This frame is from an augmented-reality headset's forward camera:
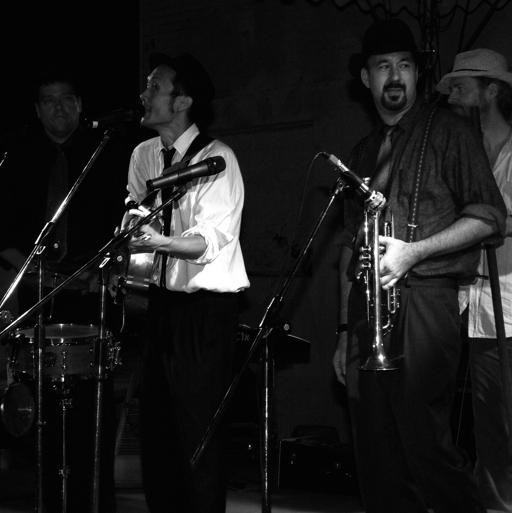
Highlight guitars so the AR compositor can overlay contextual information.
[114,161,186,315]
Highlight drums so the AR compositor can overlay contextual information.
[10,322,122,388]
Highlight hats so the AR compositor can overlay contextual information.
[349,19,438,71]
[435,48,512,95]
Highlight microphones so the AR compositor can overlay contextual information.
[146,156,226,192]
[90,104,145,129]
[325,153,388,211]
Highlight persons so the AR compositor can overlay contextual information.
[109,50,253,512]
[436,45,512,512]
[328,20,512,511]
[1,63,130,513]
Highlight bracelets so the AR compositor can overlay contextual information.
[334,322,349,335]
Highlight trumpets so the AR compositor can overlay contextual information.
[355,177,401,372]
